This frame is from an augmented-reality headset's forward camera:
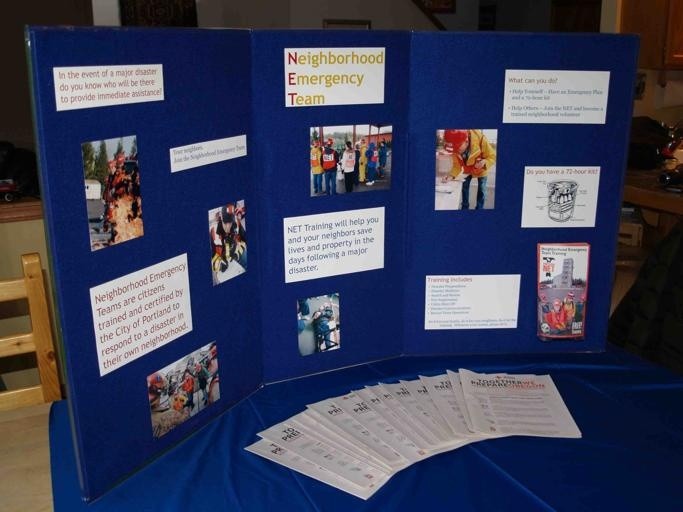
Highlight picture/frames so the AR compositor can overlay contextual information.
[119,0,198,26]
[422,0,456,14]
[322,19,371,28]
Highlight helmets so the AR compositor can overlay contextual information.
[147,369,169,406]
[359,139,366,144]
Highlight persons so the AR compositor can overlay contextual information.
[101,152,139,239]
[311,303,333,353]
[562,289,576,330]
[182,372,197,410]
[210,203,246,272]
[546,298,566,333]
[194,362,209,404]
[207,352,219,406]
[440,129,496,212]
[146,371,184,438]
[309,133,386,198]
[573,294,584,324]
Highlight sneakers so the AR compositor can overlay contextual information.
[365,180,375,186]
[313,190,324,195]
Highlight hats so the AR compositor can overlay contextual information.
[326,138,334,145]
[221,204,235,224]
[106,160,117,169]
[567,292,575,299]
[313,140,320,147]
[116,154,124,165]
[195,364,201,371]
[210,346,217,360]
[443,129,467,154]
[553,298,561,311]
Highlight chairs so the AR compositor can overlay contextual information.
[0,251,62,411]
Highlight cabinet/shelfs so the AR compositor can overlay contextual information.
[621,0,683,70]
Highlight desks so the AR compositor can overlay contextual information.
[0,197,64,392]
[622,169,683,246]
[1,343,682,511]
